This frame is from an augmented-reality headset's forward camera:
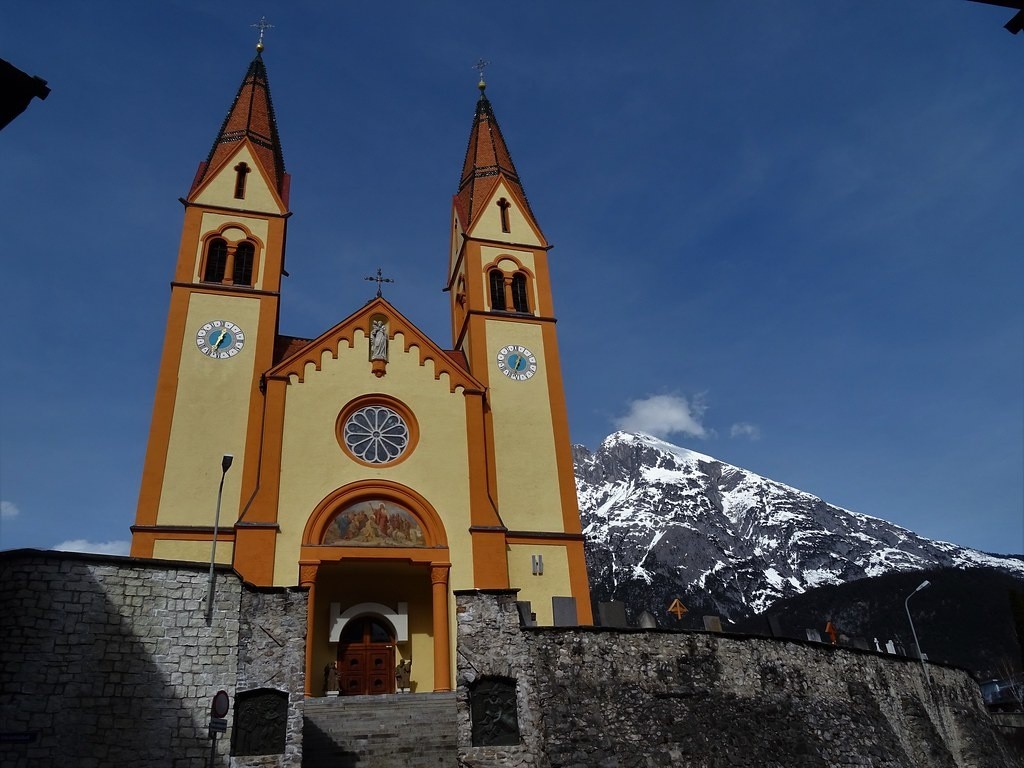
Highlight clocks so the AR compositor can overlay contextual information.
[497,345,537,380]
[196,320,245,358]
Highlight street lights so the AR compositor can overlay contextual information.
[905,579,938,700]
[203,455,233,618]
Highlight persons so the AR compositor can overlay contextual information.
[324,663,343,691]
[394,659,412,688]
[371,321,386,358]
